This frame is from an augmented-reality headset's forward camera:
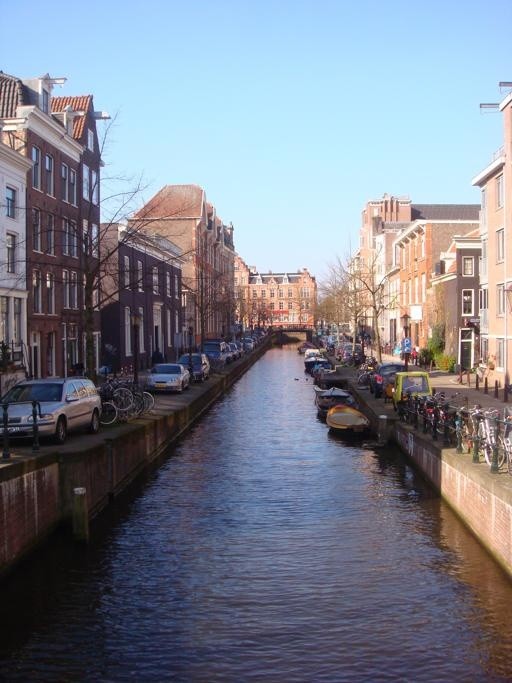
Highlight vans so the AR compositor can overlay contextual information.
[200,340,227,366]
[0,374,102,441]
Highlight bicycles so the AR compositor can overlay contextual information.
[399,390,512,475]
[357,356,379,383]
[91,373,155,425]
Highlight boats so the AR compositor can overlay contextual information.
[326,403,371,435]
[317,387,359,416]
[299,339,348,387]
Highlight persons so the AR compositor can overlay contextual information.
[154,346,164,363]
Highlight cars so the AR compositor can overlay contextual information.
[371,362,406,398]
[180,352,209,381]
[392,371,432,410]
[145,362,190,392]
[227,325,273,362]
[316,328,364,363]
